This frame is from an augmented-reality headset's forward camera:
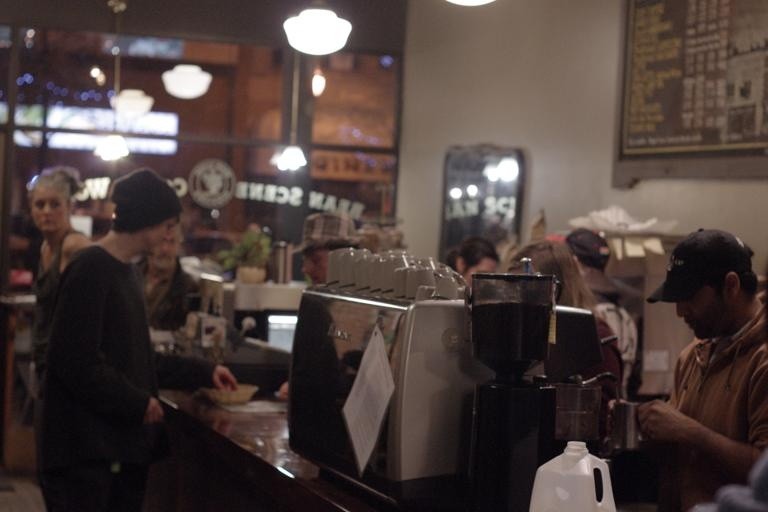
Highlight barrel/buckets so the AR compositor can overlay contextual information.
[526,439,618,512]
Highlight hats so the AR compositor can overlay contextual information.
[108,171,182,232]
[565,228,611,270]
[290,213,361,255]
[646,228,754,305]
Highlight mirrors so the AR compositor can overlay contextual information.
[438,144,523,268]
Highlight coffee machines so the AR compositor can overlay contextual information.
[458,270,557,512]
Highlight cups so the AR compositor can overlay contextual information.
[325,247,468,302]
[607,398,650,452]
